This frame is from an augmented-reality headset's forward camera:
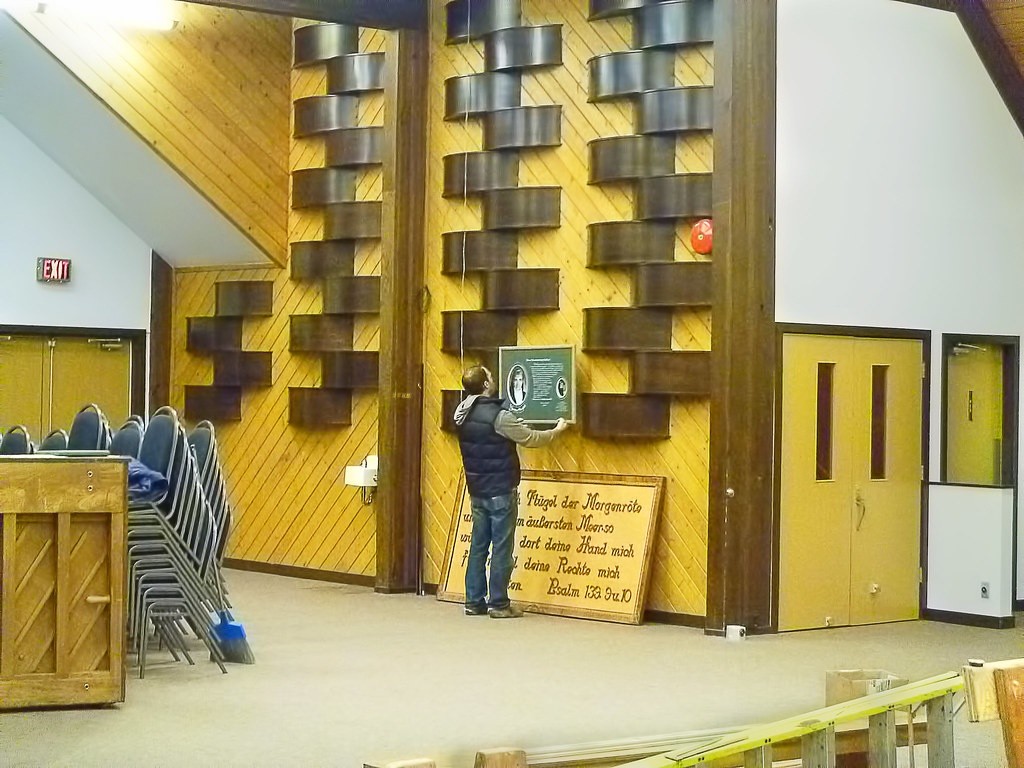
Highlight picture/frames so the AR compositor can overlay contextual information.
[435,462,668,625]
[500,344,578,428]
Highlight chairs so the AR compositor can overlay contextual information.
[0,404,236,679]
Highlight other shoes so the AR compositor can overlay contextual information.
[465,602,490,616]
[488,607,524,618]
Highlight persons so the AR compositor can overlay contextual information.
[509,369,526,403]
[453,365,570,619]
